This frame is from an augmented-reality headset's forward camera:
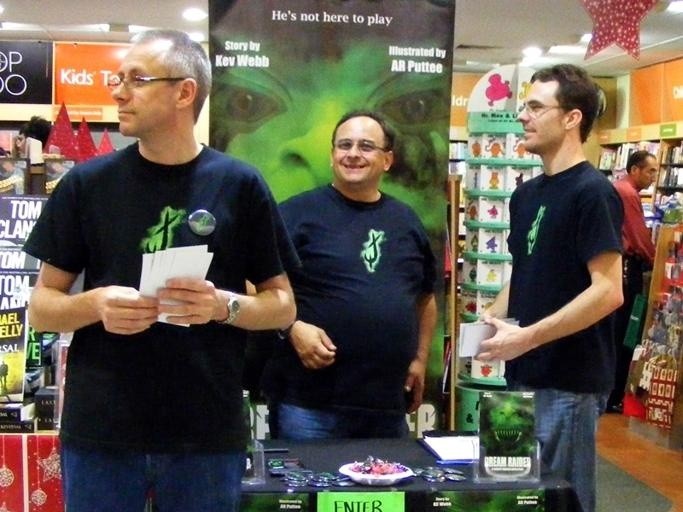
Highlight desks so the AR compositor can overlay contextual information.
[242,435,571,512]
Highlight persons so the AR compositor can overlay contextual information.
[471,62,626,512]
[21,29,297,510]
[611,149,656,348]
[268,107,440,441]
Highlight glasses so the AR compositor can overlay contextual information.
[518,101,571,115]
[333,137,386,152]
[107,69,185,91]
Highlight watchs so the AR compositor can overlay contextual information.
[215,287,241,325]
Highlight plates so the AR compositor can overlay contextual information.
[339,462,414,486]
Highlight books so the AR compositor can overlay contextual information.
[663,140,683,188]
